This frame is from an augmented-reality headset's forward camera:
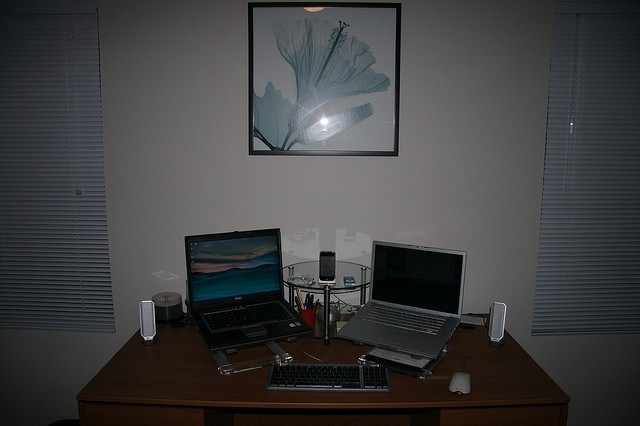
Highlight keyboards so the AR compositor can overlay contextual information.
[266,363,392,393]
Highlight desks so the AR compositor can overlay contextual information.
[283,259,372,347]
[75,312,571,425]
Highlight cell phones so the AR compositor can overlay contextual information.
[319,251,336,283]
[344,276,355,287]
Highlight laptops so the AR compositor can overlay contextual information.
[337,241,467,359]
[185,228,314,351]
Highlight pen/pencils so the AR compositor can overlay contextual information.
[295,287,319,309]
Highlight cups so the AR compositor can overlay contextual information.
[299,303,317,329]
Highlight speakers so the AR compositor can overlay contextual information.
[488,303,506,343]
[139,301,156,345]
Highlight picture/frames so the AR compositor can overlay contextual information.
[244,1,404,159]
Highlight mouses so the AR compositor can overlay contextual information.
[449,372,471,394]
